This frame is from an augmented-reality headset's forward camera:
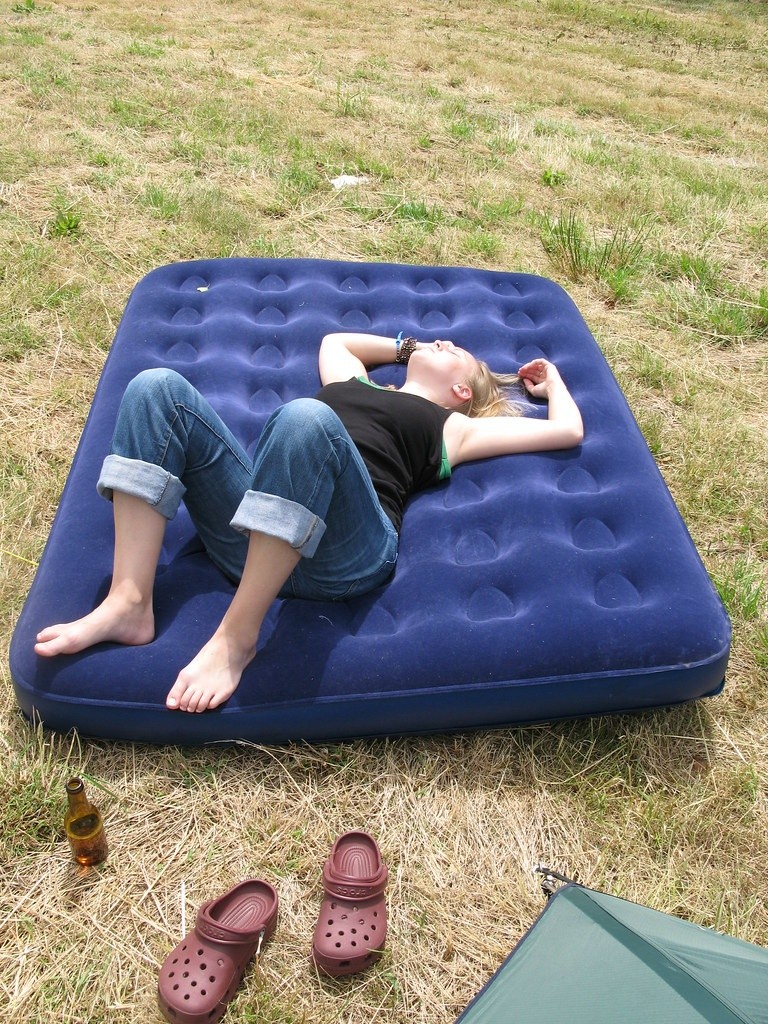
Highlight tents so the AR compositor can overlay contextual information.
[453,868,768,1024]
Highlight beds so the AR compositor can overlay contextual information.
[9,257,730,743]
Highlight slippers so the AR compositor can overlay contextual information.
[156,880,281,1022]
[311,829,390,976]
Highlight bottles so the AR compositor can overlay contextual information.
[64,777,108,866]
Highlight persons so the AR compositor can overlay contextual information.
[34,334,585,714]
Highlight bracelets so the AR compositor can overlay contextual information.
[397,331,418,364]
